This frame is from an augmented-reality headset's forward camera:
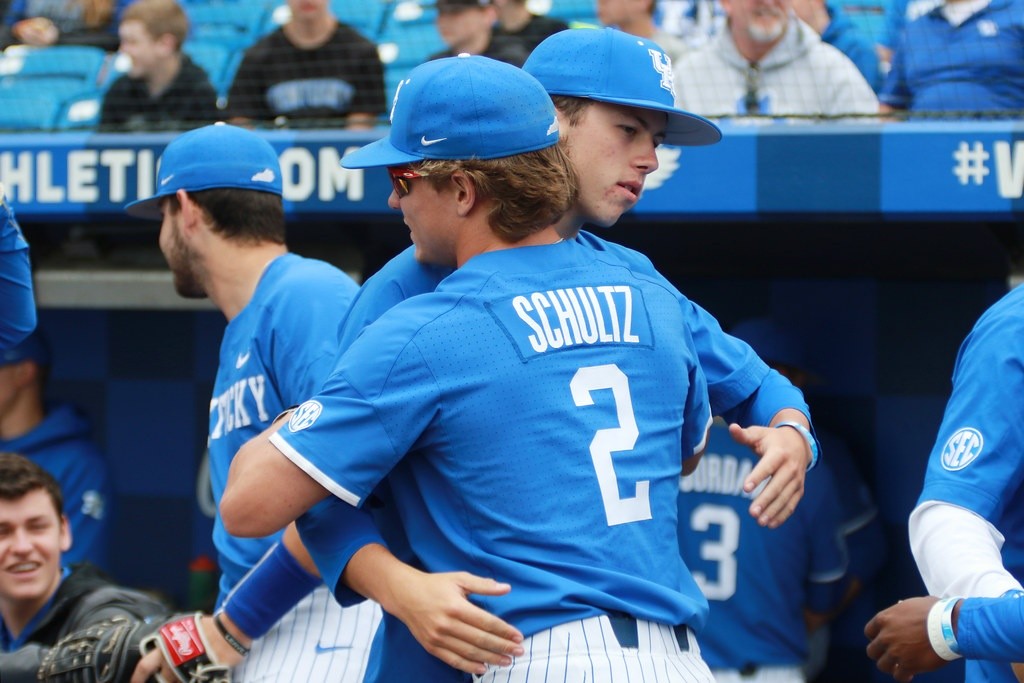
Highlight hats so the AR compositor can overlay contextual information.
[121,122,284,219]
[337,53,561,169]
[519,26,723,148]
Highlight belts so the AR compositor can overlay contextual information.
[605,613,690,653]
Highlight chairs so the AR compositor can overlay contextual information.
[0,0,893,112]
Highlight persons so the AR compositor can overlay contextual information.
[672,0,878,113]
[864,596,1024,683]
[124,123,383,683]
[0,0,119,52]
[0,451,167,683]
[790,0,1024,115]
[220,53,713,683]
[294,27,822,683]
[908,283,1024,683]
[0,180,37,345]
[0,332,112,580]
[597,0,690,64]
[100,0,220,134]
[677,319,886,682]
[222,0,387,132]
[425,0,570,68]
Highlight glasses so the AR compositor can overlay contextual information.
[384,168,455,196]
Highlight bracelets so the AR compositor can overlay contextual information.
[214,613,250,658]
[775,421,818,472]
[927,598,963,662]
